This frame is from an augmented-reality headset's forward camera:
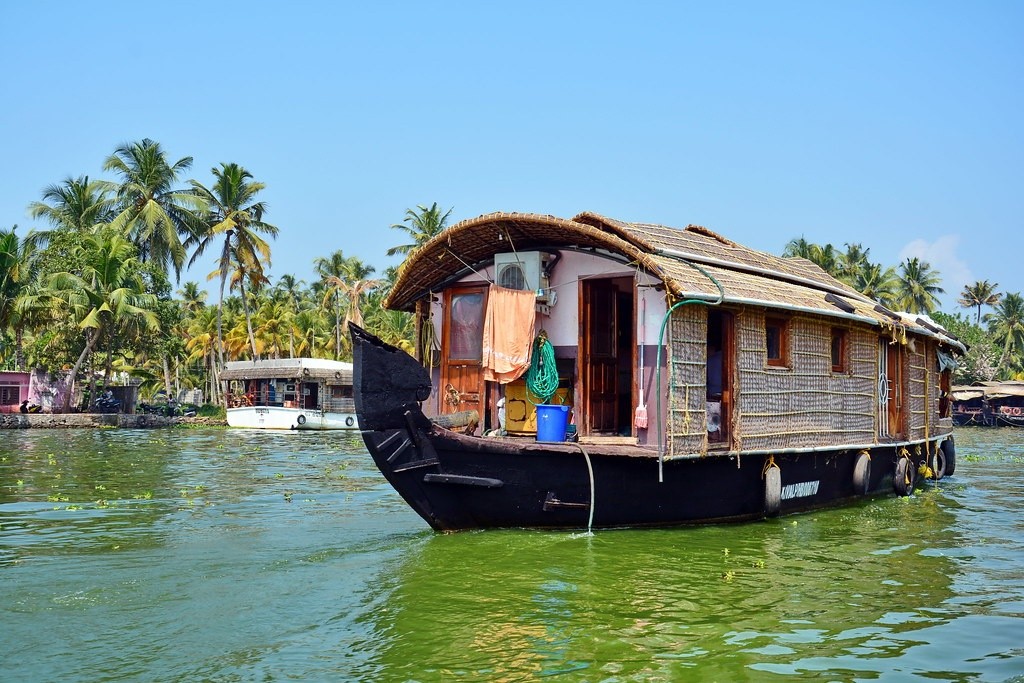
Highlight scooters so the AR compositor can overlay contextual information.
[89,397,122,413]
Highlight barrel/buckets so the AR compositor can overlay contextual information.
[535,404,571,442]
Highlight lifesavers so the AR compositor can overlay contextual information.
[764,466,782,518]
[345,417,354,426]
[239,395,248,407]
[230,397,241,408]
[1003,407,1012,415]
[939,439,956,477]
[297,415,306,425]
[852,453,871,500]
[248,394,256,406]
[893,457,916,498]
[1012,408,1021,416]
[928,447,946,480]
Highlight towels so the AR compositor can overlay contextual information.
[481,283,537,384]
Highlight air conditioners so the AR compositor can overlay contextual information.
[495,250,551,302]
[283,384,296,394]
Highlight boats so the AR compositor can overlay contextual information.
[220,357,359,430]
[347,211,971,533]
[950,380,1024,427]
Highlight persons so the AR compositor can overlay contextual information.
[940,390,956,402]
[165,393,176,418]
[100,386,116,408]
